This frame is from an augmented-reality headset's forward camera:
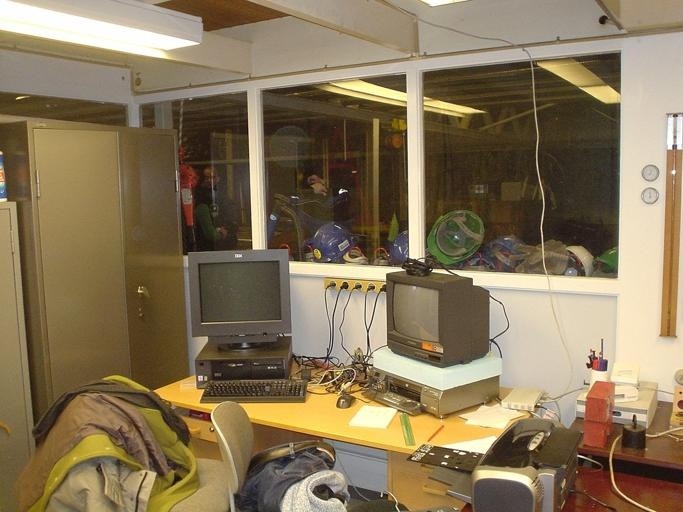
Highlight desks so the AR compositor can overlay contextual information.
[563,456,683,511]
[147,369,543,512]
[566,398,681,471]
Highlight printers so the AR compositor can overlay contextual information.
[406,418,585,512]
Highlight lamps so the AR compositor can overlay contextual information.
[315,81,488,119]
[1,0,204,59]
[538,59,620,105]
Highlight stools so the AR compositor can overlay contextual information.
[136,456,231,512]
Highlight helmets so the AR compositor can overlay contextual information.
[566,244,596,279]
[597,244,618,273]
[427,207,487,265]
[489,234,531,273]
[392,229,409,263]
[314,222,353,260]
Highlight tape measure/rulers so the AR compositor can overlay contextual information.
[400,413,416,445]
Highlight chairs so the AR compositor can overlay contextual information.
[210,400,255,512]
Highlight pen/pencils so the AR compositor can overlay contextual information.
[428,425,445,442]
[586,339,608,371]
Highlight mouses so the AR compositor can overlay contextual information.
[336,396,355,409]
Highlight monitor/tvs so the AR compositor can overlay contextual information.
[386,271,489,368]
[188,249,292,350]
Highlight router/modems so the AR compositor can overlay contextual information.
[501,388,544,411]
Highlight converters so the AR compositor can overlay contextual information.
[301,369,311,380]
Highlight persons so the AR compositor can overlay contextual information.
[190,164,239,251]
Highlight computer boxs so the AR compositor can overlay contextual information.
[361,349,503,419]
[194,336,292,389]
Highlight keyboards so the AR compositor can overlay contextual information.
[200,379,308,403]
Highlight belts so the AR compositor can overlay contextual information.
[249,436,340,470]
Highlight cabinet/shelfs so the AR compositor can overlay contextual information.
[0,200,38,512]
[0,119,185,419]
